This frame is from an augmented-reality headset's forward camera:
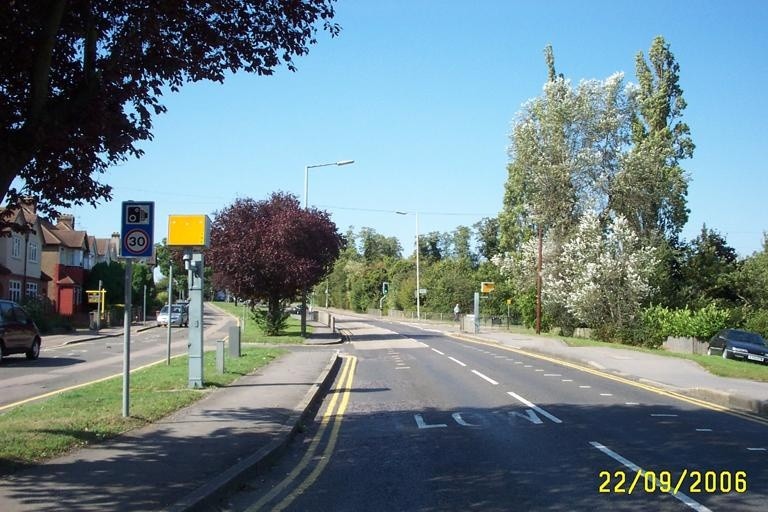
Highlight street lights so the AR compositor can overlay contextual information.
[297,159,355,338]
[394,210,421,320]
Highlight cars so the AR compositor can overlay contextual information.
[0,299,42,361]
[156,303,188,329]
[706,327,766,368]
[212,289,309,316]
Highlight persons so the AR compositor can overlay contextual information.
[452,304,461,322]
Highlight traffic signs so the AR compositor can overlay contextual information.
[123,228,151,255]
